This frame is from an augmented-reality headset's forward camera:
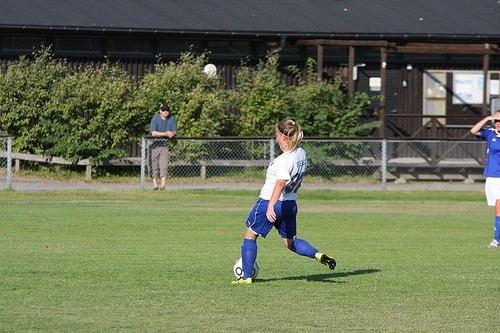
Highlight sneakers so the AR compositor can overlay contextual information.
[488,240,500,248]
[315,252,336,270]
[231,278,253,283]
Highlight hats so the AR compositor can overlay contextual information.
[159,103,169,109]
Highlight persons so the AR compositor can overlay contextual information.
[470,109,500,247]
[231,120,337,284]
[149,103,177,190]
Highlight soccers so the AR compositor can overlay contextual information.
[233,257,259,281]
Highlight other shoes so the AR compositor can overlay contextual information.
[154,186,158,190]
[160,186,164,190]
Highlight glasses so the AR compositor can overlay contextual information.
[161,109,169,111]
